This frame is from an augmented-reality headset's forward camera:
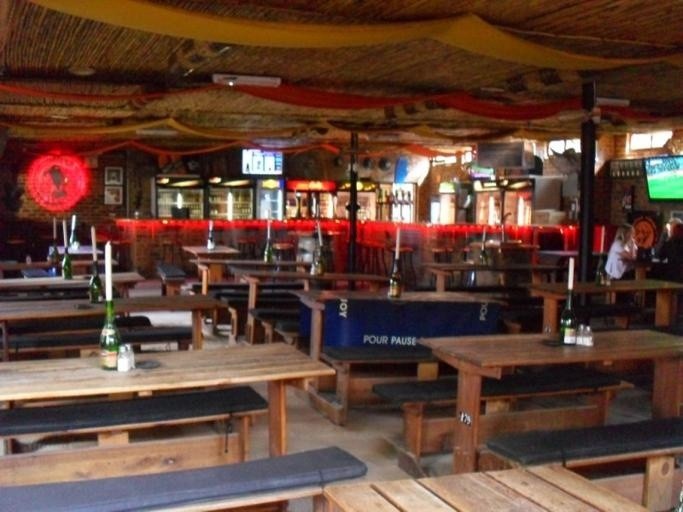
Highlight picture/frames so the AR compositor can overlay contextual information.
[103,165,123,186]
[102,185,124,206]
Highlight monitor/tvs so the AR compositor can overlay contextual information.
[241,148,285,177]
[642,155,683,203]
[476,142,524,169]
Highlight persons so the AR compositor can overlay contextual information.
[657,218,683,280]
[605,223,636,281]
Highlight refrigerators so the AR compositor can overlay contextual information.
[209,176,253,219]
[151,175,205,218]
[287,180,336,219]
[255,178,285,219]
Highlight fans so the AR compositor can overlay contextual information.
[544,147,582,195]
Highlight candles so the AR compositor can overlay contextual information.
[51,215,58,241]
[265,219,271,240]
[316,221,323,246]
[59,216,69,248]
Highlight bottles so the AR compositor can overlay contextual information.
[309,245,325,276]
[88,263,101,303]
[389,261,403,301]
[556,288,579,346]
[48,228,82,280]
[206,230,216,250]
[596,255,615,289]
[478,244,487,264]
[264,240,275,263]
[609,159,643,177]
[159,190,331,219]
[118,345,139,373]
[99,299,118,369]
[579,324,595,347]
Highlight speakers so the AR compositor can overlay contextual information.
[583,82,595,111]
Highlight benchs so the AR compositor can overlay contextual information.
[1,234,682,512]
